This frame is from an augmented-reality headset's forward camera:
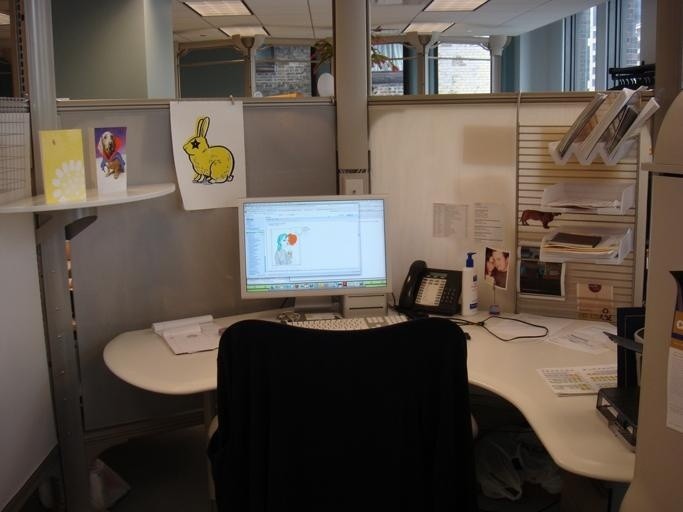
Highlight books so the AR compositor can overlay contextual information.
[556,88,659,162]
[541,226,627,261]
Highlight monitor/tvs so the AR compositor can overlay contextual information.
[239,195,388,319]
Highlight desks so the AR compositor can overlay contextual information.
[96,300,636,488]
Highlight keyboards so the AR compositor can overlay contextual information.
[286,315,406,331]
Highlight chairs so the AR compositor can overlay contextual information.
[208,315,485,508]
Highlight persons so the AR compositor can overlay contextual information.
[485,248,510,286]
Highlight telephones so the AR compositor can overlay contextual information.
[399,260,462,316]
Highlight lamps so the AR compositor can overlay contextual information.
[408,31,440,56]
[481,33,507,56]
[231,30,265,57]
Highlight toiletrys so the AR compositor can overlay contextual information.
[462,252,479,317]
